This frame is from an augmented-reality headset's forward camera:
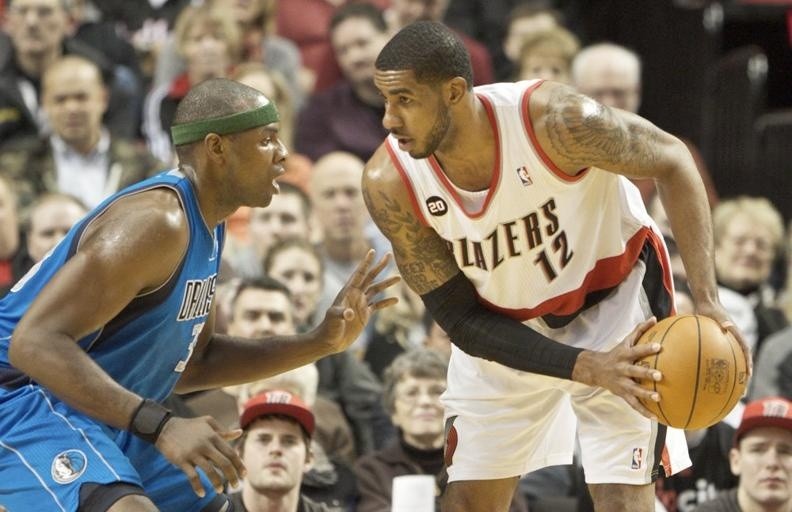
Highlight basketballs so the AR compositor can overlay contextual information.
[633,315,745,429]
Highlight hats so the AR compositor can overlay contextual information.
[239,389,315,436]
[734,396,792,450]
